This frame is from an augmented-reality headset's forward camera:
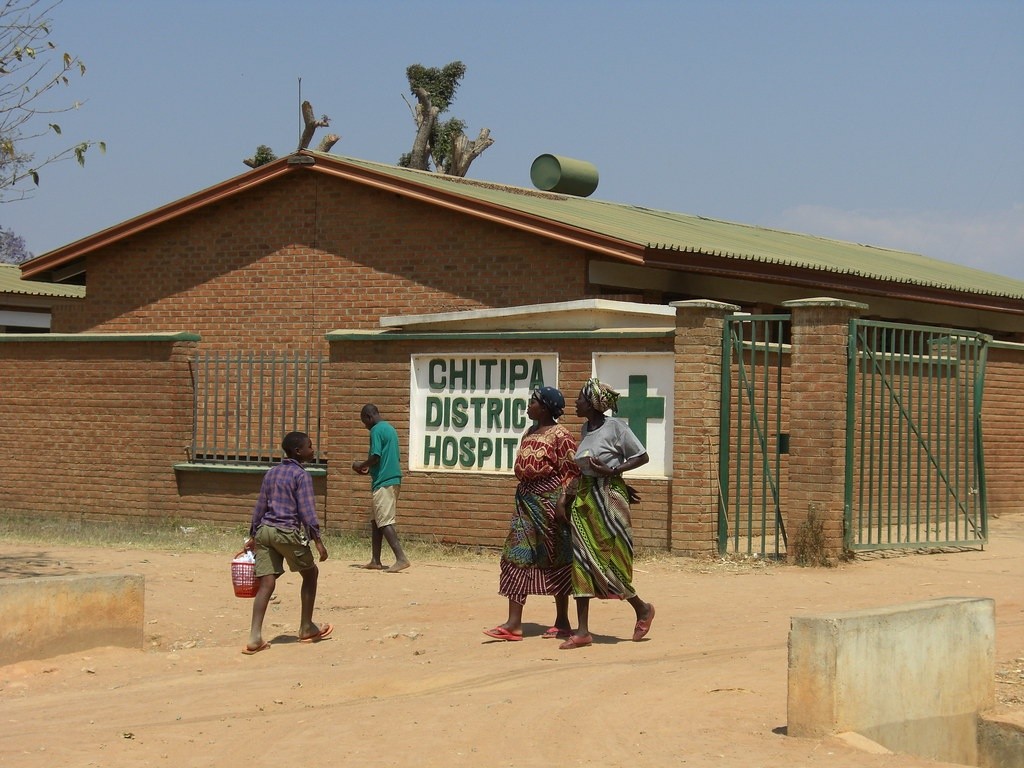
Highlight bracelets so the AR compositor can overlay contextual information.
[611,465,617,475]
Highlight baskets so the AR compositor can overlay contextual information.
[231,548,260,597]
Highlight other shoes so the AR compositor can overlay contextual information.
[560,631,593,649]
[633,603,655,641]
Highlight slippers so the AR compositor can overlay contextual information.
[483,625,523,641]
[298,621,334,642]
[242,639,271,655]
[543,625,573,638]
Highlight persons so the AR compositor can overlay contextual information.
[559,378,655,649]
[352,403,411,572]
[242,432,334,654]
[482,387,573,640]
